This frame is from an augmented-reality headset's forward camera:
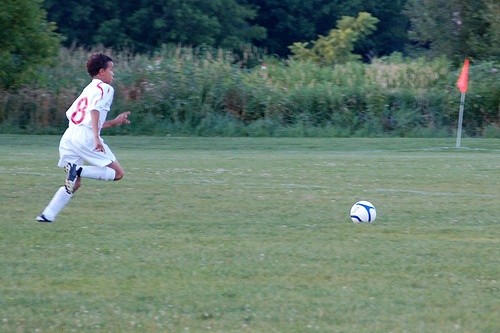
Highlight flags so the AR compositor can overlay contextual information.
[457,59,470,93]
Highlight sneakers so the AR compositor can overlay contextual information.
[64,162,78,194]
[36,214,52,223]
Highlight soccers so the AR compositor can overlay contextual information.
[349,200,377,225]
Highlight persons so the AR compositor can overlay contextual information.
[34,53,131,224]
